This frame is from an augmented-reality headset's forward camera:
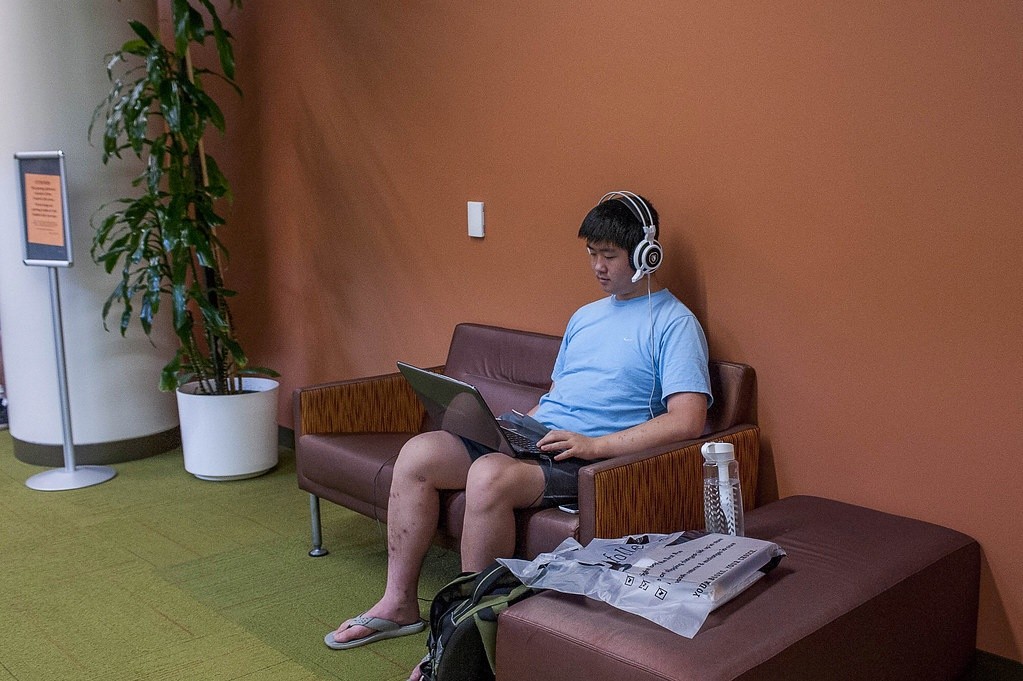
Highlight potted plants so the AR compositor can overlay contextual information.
[86,0,281,482]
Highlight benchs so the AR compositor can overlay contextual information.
[293,323,760,561]
[495,495,980,681]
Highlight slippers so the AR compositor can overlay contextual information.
[324,612,426,650]
[406,651,432,681]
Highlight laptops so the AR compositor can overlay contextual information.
[396,361,563,458]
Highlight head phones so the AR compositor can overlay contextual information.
[598,190,664,283]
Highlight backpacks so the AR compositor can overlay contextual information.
[427,562,559,681]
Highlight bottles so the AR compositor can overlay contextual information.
[700,440,744,537]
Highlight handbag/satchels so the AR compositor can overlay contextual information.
[494,531,787,638]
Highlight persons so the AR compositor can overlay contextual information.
[324,190,713,681]
